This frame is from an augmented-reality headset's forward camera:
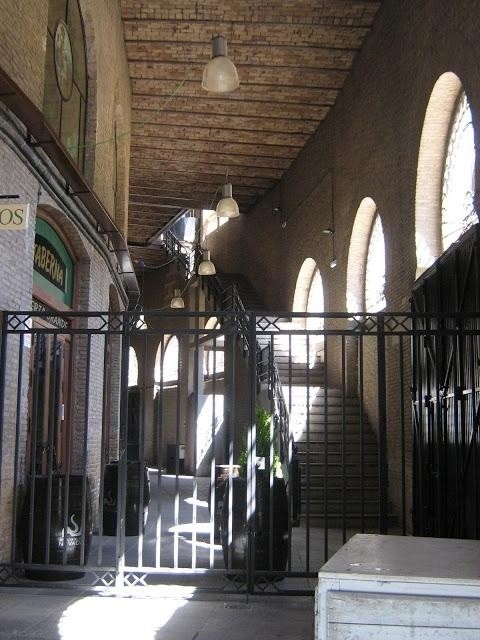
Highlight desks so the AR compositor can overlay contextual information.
[312,532,480,640]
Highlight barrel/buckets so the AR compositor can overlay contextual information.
[221,478,288,582]
[17,474,92,581]
[208,464,241,538]
[99,462,150,536]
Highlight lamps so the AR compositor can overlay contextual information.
[214,171,240,219]
[201,23,240,93]
[132,262,149,330]
[170,257,186,310]
[196,239,217,276]
